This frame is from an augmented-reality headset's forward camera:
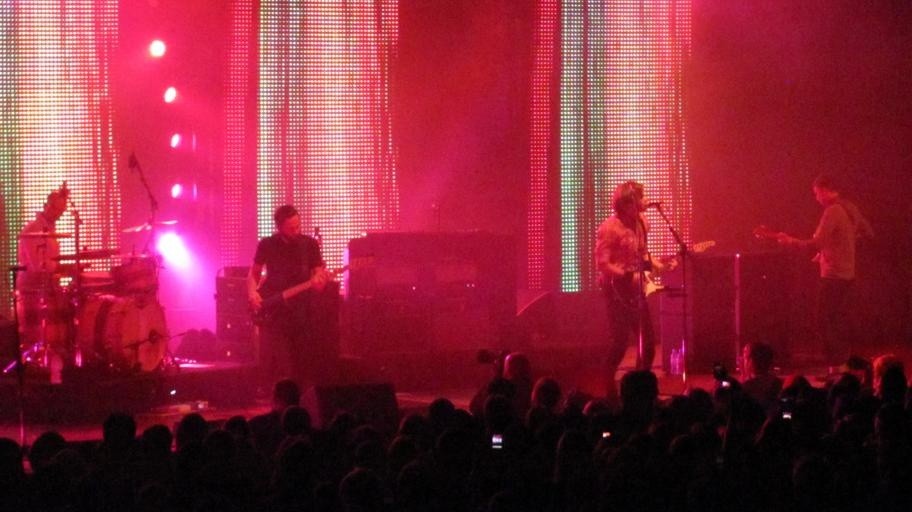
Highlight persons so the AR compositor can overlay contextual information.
[773,175,879,363]
[246,204,338,379]
[17,192,69,334]
[1,340,912,512]
[595,180,676,391]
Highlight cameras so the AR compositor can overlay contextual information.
[475,348,510,368]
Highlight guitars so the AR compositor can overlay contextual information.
[250,259,353,327]
[753,225,881,281]
[603,241,715,311]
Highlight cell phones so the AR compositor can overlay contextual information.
[603,431,611,438]
[781,410,791,422]
[491,430,502,449]
[713,362,727,378]
[721,379,730,388]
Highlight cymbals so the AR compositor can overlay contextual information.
[111,251,153,258]
[92,248,120,255]
[122,219,177,233]
[17,231,70,238]
[51,248,111,260]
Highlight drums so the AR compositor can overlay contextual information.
[80,292,168,375]
[70,271,115,299]
[110,263,159,293]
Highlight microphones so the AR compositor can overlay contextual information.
[313,224,322,236]
[645,201,663,210]
